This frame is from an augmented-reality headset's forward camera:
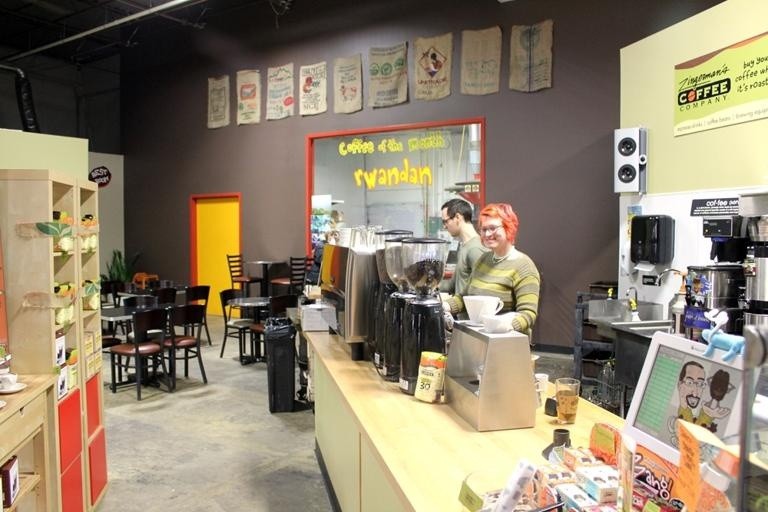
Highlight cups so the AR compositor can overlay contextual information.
[350,224,386,251]
[554,429,571,448]
[556,377,581,423]
[0,371,19,390]
[463,295,504,323]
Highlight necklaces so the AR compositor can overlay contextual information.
[492,245,514,264]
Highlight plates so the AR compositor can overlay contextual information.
[0,400,7,408]
[0,382,29,394]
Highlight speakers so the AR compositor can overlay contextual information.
[611,127,647,196]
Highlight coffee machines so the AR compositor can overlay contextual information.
[370,228,451,395]
[739,192,768,314]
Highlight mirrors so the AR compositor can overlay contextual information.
[305,117,485,269]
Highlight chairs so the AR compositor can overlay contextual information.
[101,252,305,400]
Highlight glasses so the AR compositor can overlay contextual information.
[479,224,503,236]
[442,217,451,226]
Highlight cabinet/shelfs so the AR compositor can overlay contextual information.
[0,168,109,512]
[0,373,54,512]
[574,281,617,397]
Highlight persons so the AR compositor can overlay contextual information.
[444,202,541,347]
[439,200,489,295]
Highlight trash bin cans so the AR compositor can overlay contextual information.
[263,317,297,413]
